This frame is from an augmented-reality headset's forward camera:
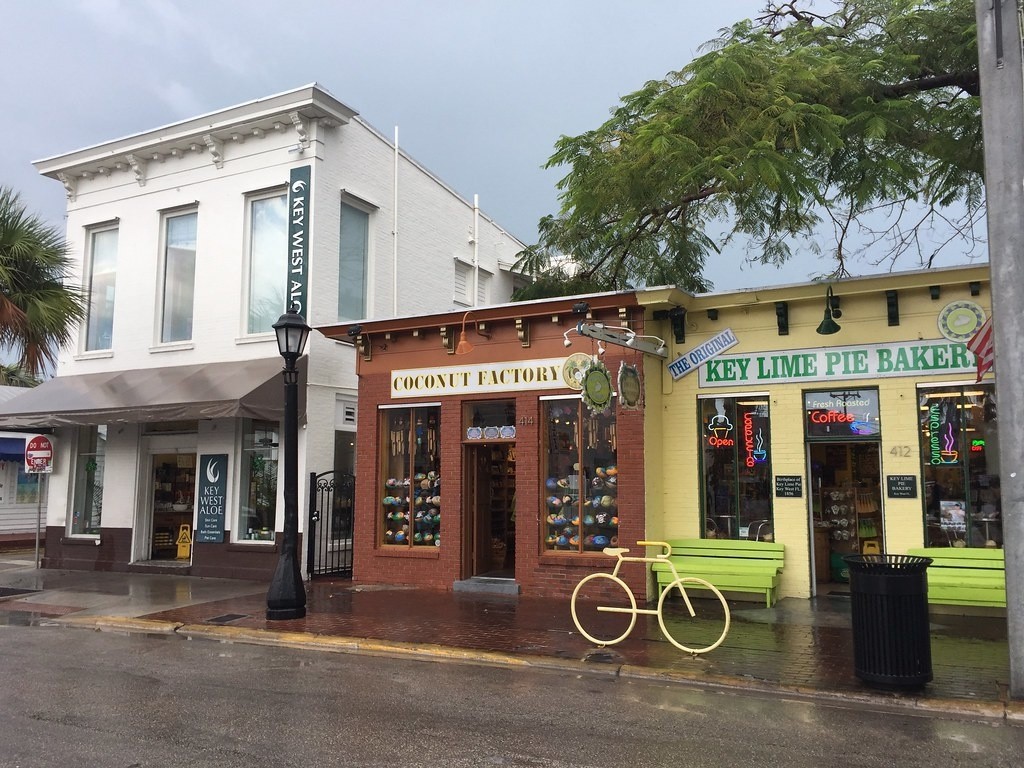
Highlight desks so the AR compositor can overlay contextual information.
[971,517,999,541]
[709,514,744,539]
[927,524,958,547]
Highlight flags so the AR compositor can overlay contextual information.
[966,315,993,384]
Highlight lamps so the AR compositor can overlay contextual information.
[347,325,365,336]
[563,323,666,356]
[817,285,841,336]
[572,302,592,314]
[455,312,492,355]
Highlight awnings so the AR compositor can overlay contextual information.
[0,354,307,430]
[0,437,26,463]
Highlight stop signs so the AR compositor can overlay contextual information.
[25,435,54,474]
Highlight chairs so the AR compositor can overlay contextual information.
[746,519,772,541]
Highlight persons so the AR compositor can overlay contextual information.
[975,478,1004,549]
[948,502,965,522]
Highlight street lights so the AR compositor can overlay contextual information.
[266,302,312,621]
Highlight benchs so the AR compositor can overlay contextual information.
[907,547,1006,609]
[651,539,785,608]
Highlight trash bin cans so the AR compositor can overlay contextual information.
[841,554,935,692]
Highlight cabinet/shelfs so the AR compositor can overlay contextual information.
[548,491,578,546]
[385,486,410,540]
[584,487,618,548]
[855,488,884,556]
[413,487,441,542]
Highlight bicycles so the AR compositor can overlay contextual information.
[571,541,730,653]
